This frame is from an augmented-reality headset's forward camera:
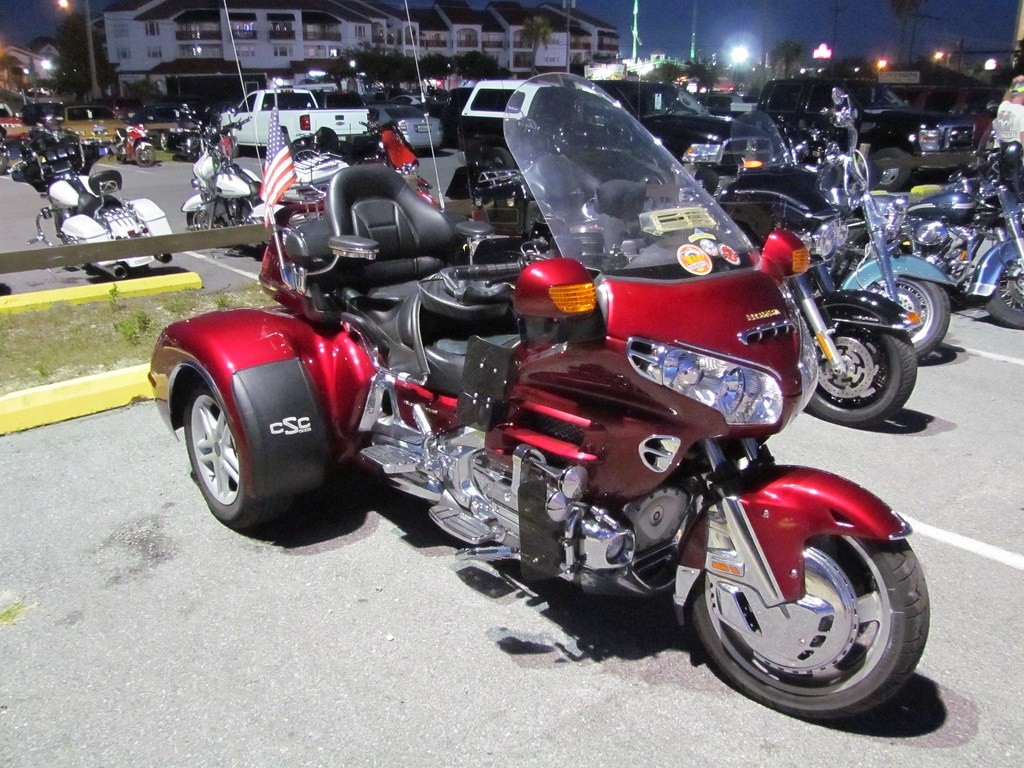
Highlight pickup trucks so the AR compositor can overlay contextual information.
[220,88,370,157]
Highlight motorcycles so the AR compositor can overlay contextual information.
[148,72,930,721]
[0,103,239,176]
[180,105,436,259]
[8,138,174,278]
[472,87,1024,430]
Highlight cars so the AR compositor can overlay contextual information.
[0,103,131,141]
[356,105,443,149]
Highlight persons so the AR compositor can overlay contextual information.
[975,33,1024,174]
[684,60,714,98]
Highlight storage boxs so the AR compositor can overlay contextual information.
[59,213,113,269]
[127,195,174,236]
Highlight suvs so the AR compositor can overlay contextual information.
[757,78,976,192]
[457,79,773,172]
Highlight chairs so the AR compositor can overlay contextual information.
[326,165,456,302]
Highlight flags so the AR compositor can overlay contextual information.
[258,106,297,230]
